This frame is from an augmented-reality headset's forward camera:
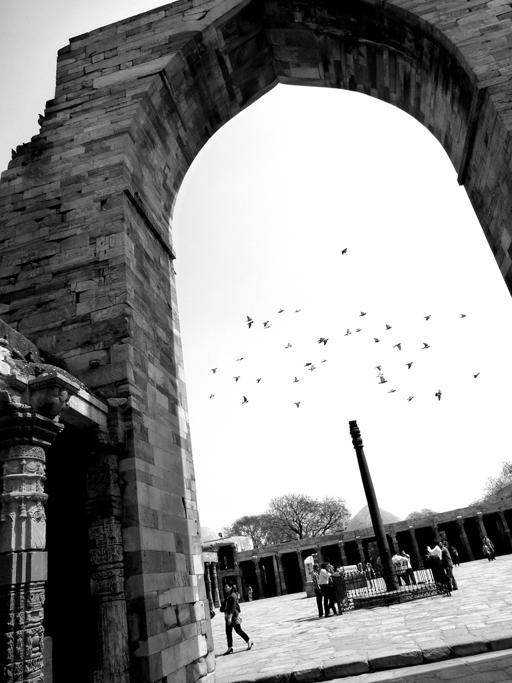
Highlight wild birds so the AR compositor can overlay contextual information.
[459,314,466,318]
[246,316,270,329]
[278,308,301,313]
[210,357,262,404]
[285,338,331,408]
[473,372,480,379]
[360,312,367,316]
[342,248,347,255]
[374,315,442,403]
[344,328,362,336]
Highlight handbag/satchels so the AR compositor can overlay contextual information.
[219,601,226,612]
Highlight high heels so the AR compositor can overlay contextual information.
[247,642,254,650]
[223,648,233,655]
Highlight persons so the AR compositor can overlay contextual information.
[230,583,241,604]
[480,535,496,560]
[481,543,494,562]
[220,582,255,655]
[311,538,461,616]
